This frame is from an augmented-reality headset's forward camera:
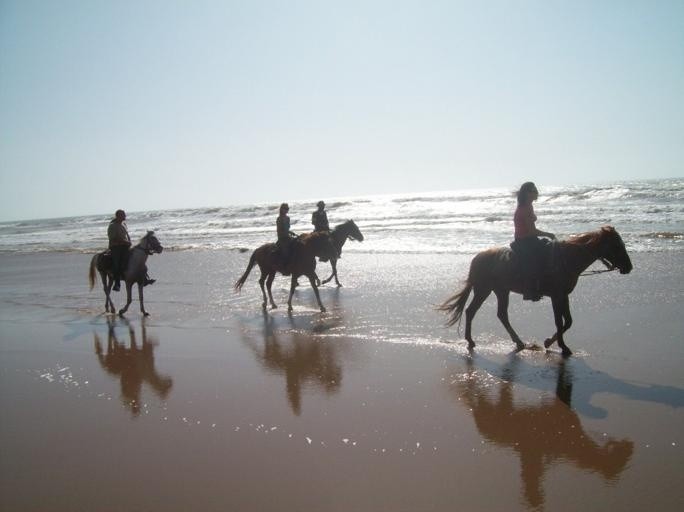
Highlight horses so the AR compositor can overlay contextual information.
[297,218,364,285]
[88,231,163,317]
[437,225,634,355]
[233,229,339,312]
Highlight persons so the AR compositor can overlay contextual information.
[312,201,329,231]
[276,203,295,243]
[107,210,155,291]
[515,183,555,300]
